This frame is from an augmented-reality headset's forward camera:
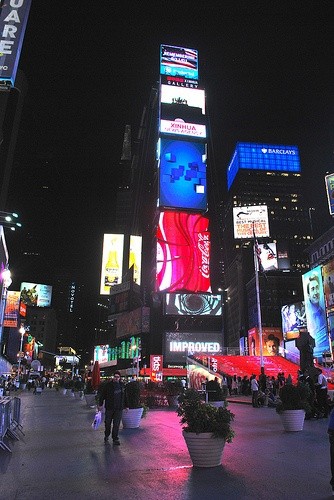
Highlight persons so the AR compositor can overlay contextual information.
[98,371,127,444]
[282,275,330,353]
[32,375,46,395]
[264,334,282,356]
[251,339,256,356]
[221,368,329,419]
[258,244,278,269]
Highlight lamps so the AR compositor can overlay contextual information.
[0,210,21,231]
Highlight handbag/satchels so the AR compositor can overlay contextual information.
[91,411,101,430]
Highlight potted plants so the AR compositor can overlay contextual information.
[276,381,313,431]
[176,396,237,468]
[167,381,180,407]
[121,382,148,429]
[58,379,97,406]
[207,380,225,408]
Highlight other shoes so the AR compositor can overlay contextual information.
[113,440,121,445]
[104,437,108,441]
